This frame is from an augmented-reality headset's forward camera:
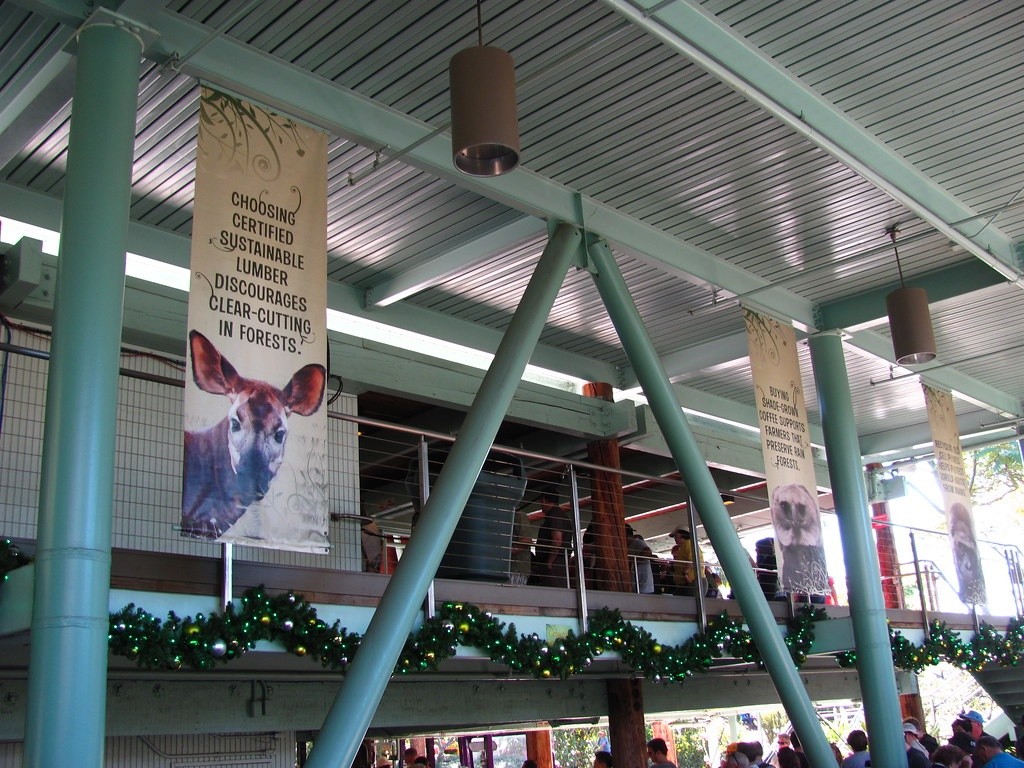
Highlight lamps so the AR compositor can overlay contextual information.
[449,0,520,178]
[885,229,937,367]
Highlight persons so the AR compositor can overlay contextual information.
[595,731,610,752]
[930,711,1024,768]
[728,546,760,600]
[522,759,537,768]
[404,747,432,768]
[375,755,391,768]
[718,741,776,768]
[778,730,810,768]
[704,560,723,599]
[823,574,839,606]
[668,524,694,584]
[625,523,656,594]
[901,716,939,768]
[842,730,870,768]
[593,751,614,768]
[535,486,572,588]
[506,509,533,585]
[646,737,677,768]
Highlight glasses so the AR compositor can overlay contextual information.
[777,742,790,746]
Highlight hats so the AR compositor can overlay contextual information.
[598,731,604,734]
[669,525,689,537]
[959,710,984,723]
[904,723,917,735]
